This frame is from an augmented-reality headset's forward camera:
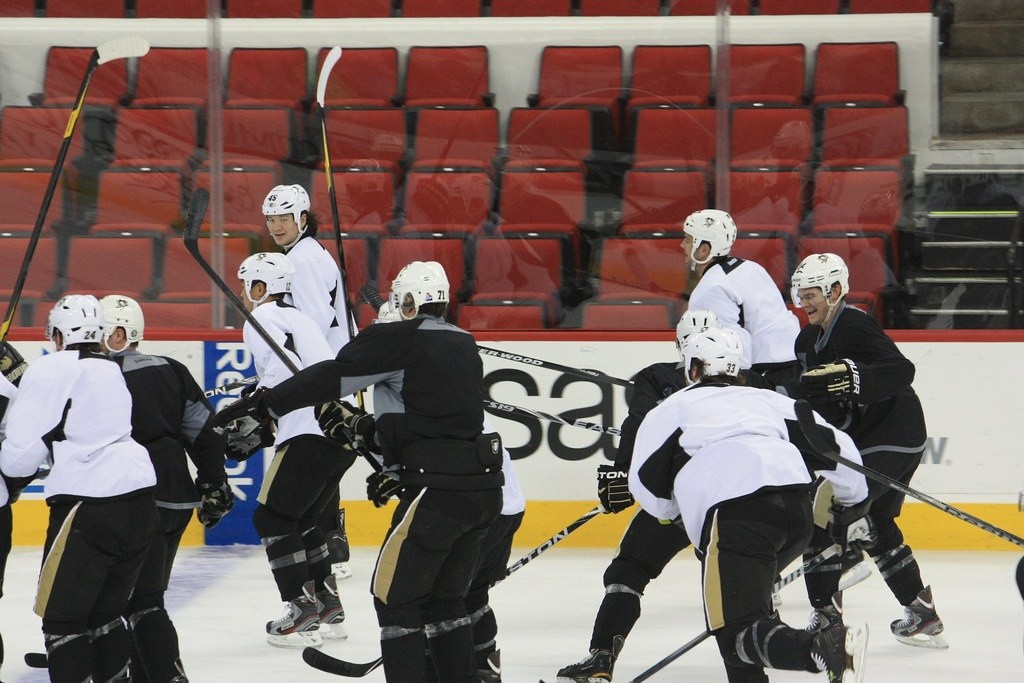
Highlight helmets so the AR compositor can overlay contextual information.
[676,308,721,350]
[683,209,737,265]
[790,252,849,308]
[682,328,743,384]
[100,295,144,352]
[262,184,311,233]
[48,294,105,350]
[391,260,450,319]
[237,253,293,304]
[378,301,402,323]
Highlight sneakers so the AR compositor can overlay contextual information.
[839,542,873,592]
[804,590,844,634]
[891,585,949,649]
[556,634,625,683]
[812,621,869,683]
[477,649,501,683]
[265,573,348,650]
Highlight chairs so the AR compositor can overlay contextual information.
[0,0,933,340]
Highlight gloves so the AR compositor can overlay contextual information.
[366,464,407,508]
[314,400,382,456]
[825,495,878,559]
[209,386,275,461]
[195,473,234,530]
[801,359,861,406]
[598,464,636,513]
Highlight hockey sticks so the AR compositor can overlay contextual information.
[1,36,152,346]
[24,652,48,669]
[314,43,367,415]
[302,503,607,681]
[180,187,404,499]
[791,396,1024,550]
[480,398,622,439]
[359,281,635,390]
[629,525,867,683]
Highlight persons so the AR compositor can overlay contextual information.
[225,183,526,636]
[0,294,234,683]
[210,260,506,683]
[680,209,802,400]
[559,252,945,683]
